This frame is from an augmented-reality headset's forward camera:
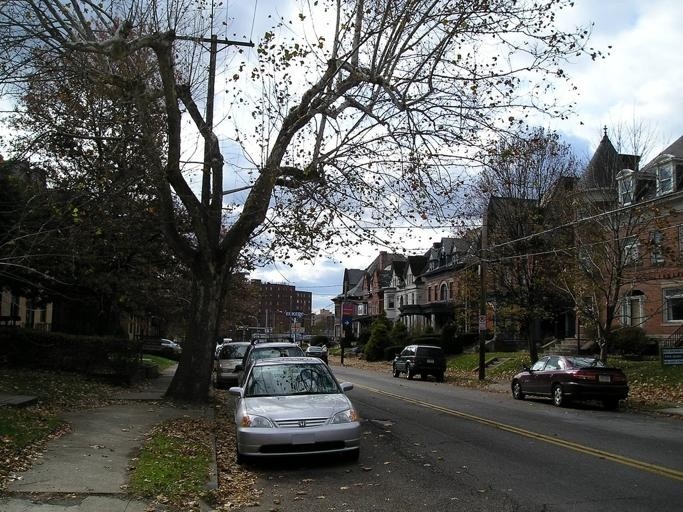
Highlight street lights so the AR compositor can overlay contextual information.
[249,315,260,331]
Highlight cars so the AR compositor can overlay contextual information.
[214,341,360,464]
[161,339,181,353]
[511,355,629,410]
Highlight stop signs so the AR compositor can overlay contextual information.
[344,303,354,316]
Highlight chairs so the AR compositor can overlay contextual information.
[249,371,279,395]
[293,370,320,392]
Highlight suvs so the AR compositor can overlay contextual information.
[392,345,446,382]
